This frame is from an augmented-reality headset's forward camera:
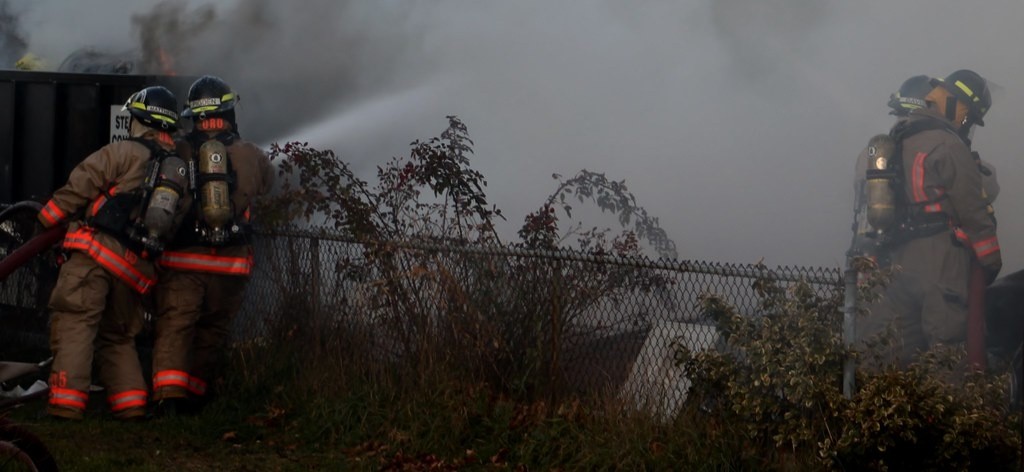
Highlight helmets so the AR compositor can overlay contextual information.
[930,67,994,128]
[181,75,241,116]
[128,86,181,132]
[889,75,944,114]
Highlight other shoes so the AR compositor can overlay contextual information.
[157,384,202,413]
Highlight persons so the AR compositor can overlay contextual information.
[29,85,185,420]
[845,67,1001,390]
[151,76,274,423]
